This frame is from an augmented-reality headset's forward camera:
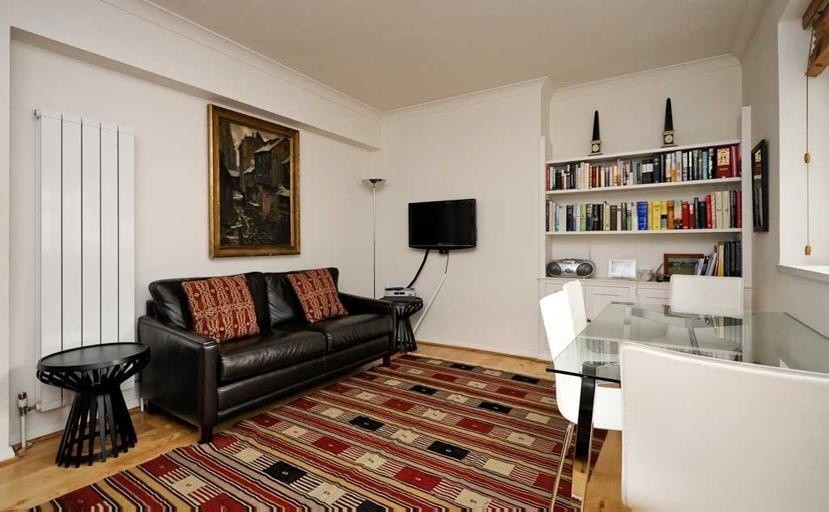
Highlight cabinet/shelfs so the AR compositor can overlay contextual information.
[539,284,635,358]
[635,284,670,319]
[543,139,742,283]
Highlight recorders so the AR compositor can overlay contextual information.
[547,259,596,278]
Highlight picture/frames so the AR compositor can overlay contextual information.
[663,253,705,276]
[750,138,771,233]
[608,258,637,280]
[205,100,302,260]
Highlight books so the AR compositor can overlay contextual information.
[544,145,741,192]
[545,187,743,232]
[696,239,742,276]
[700,313,743,345]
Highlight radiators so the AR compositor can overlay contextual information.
[30,107,136,411]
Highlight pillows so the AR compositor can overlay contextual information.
[181,275,262,341]
[284,266,349,325]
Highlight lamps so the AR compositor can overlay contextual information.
[362,178,386,299]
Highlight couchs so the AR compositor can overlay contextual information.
[137,264,397,444]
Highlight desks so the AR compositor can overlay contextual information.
[546,300,827,503]
[35,340,152,468]
[377,295,423,358]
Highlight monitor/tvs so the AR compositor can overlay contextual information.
[408,199,477,248]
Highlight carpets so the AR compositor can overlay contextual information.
[0,351,609,512]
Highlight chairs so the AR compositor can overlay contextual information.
[563,277,619,363]
[669,272,744,322]
[539,279,621,512]
[617,340,829,512]
